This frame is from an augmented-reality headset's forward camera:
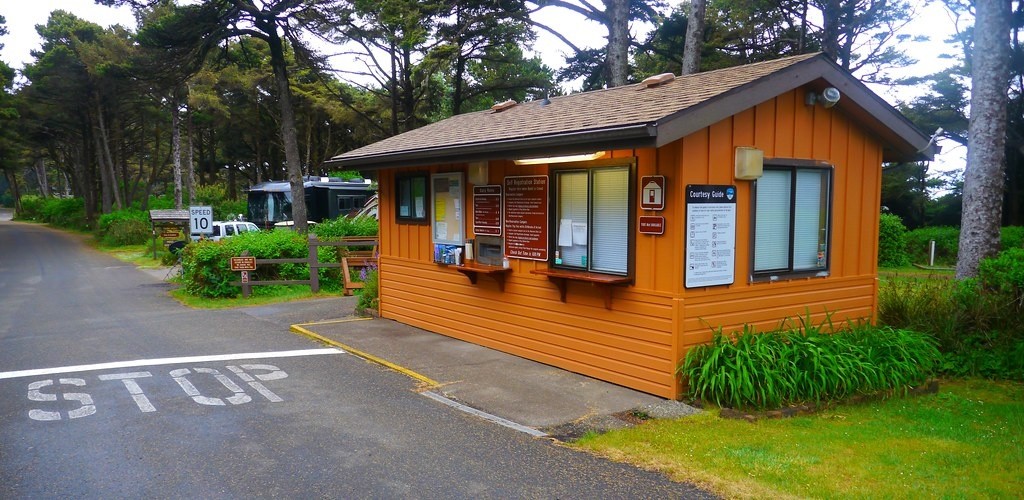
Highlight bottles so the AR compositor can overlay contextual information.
[502,255,509,268]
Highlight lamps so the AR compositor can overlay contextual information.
[513,151,605,165]
[805,87,840,108]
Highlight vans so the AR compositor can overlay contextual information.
[190,221,261,241]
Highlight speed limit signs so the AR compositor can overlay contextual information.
[189,206,213,234]
